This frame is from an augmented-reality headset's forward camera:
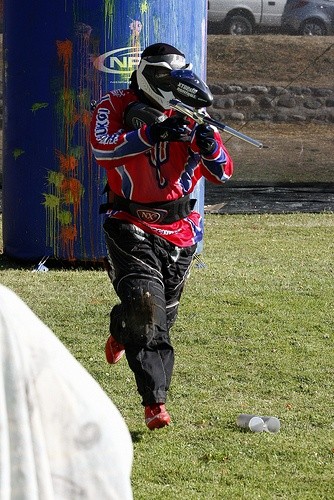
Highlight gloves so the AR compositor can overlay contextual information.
[145,116,192,143]
[195,123,216,156]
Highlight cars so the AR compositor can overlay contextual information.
[209,0,334,38]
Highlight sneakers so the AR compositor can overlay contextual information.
[105,334,125,364]
[144,404,170,430]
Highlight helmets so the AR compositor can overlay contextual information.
[137,43,185,109]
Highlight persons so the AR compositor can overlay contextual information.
[89,43,234,431]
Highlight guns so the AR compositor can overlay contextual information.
[122,69,264,149]
